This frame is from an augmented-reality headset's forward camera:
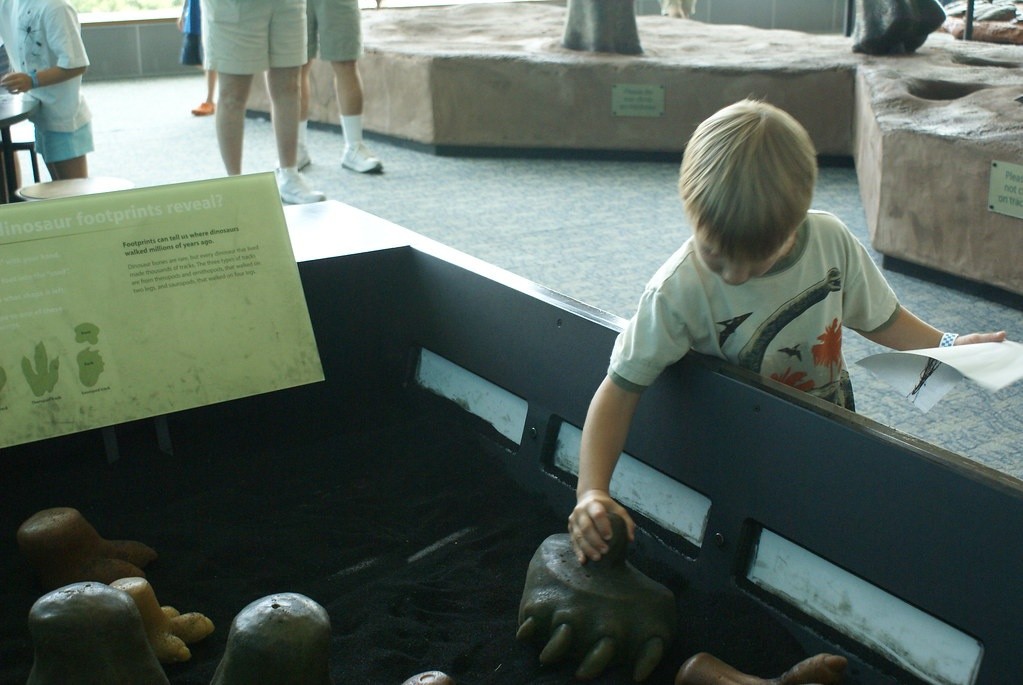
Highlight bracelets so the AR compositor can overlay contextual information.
[28,71,40,88]
[939,332,959,347]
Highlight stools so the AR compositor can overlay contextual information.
[15,178,135,202]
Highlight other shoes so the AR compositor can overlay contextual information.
[193,103,214,116]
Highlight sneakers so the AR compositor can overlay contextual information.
[274,153,311,173]
[340,144,383,174]
[276,175,326,204]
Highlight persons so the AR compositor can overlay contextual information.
[178,0,383,202]
[0,0,93,181]
[568,99,1006,566]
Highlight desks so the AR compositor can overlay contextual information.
[0,91,39,204]
